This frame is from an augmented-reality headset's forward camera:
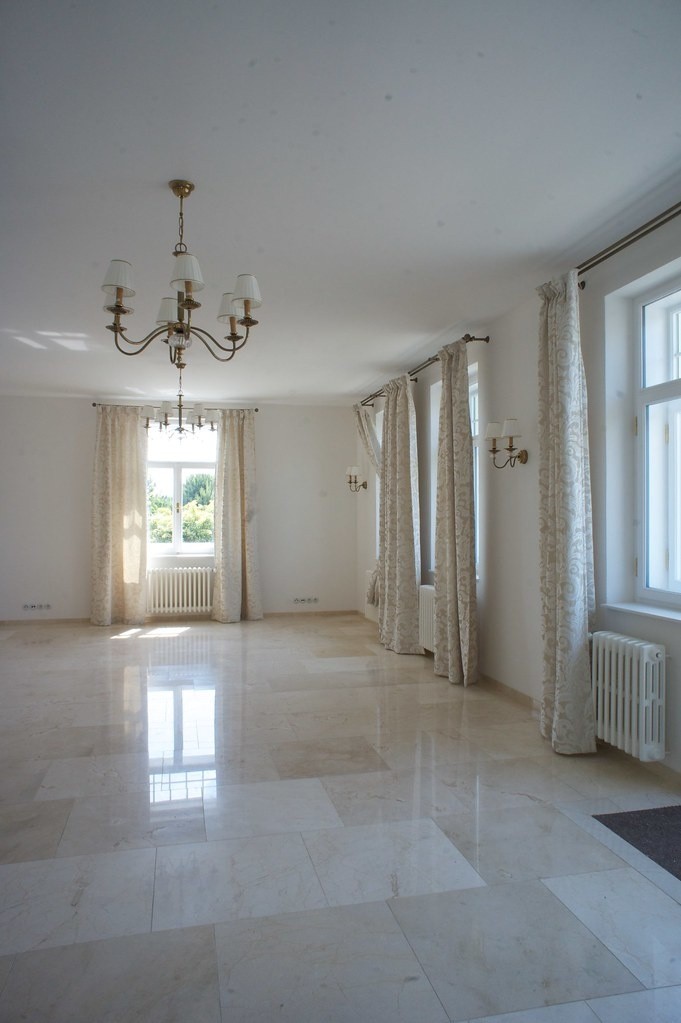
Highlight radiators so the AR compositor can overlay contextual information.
[415,583,436,651]
[585,629,667,761]
[144,567,215,614]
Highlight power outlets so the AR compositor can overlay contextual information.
[23,603,51,610]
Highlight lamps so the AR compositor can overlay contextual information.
[345,465,369,492]
[484,418,529,469]
[95,176,264,432]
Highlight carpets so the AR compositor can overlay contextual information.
[591,805,680,889]
